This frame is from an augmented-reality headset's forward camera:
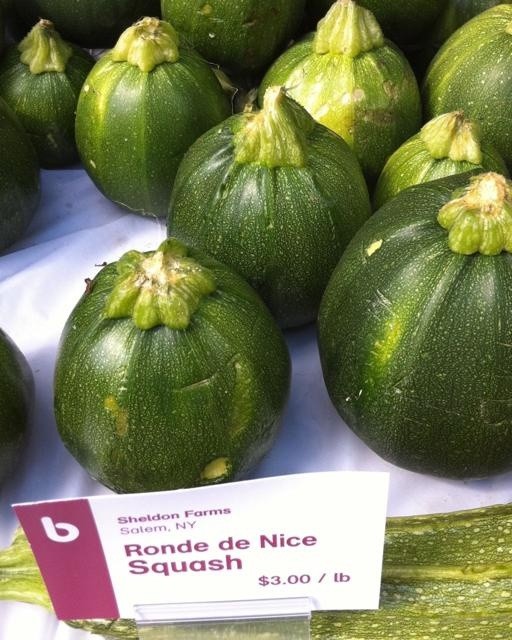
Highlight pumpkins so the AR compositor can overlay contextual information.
[0,1,512,494]
